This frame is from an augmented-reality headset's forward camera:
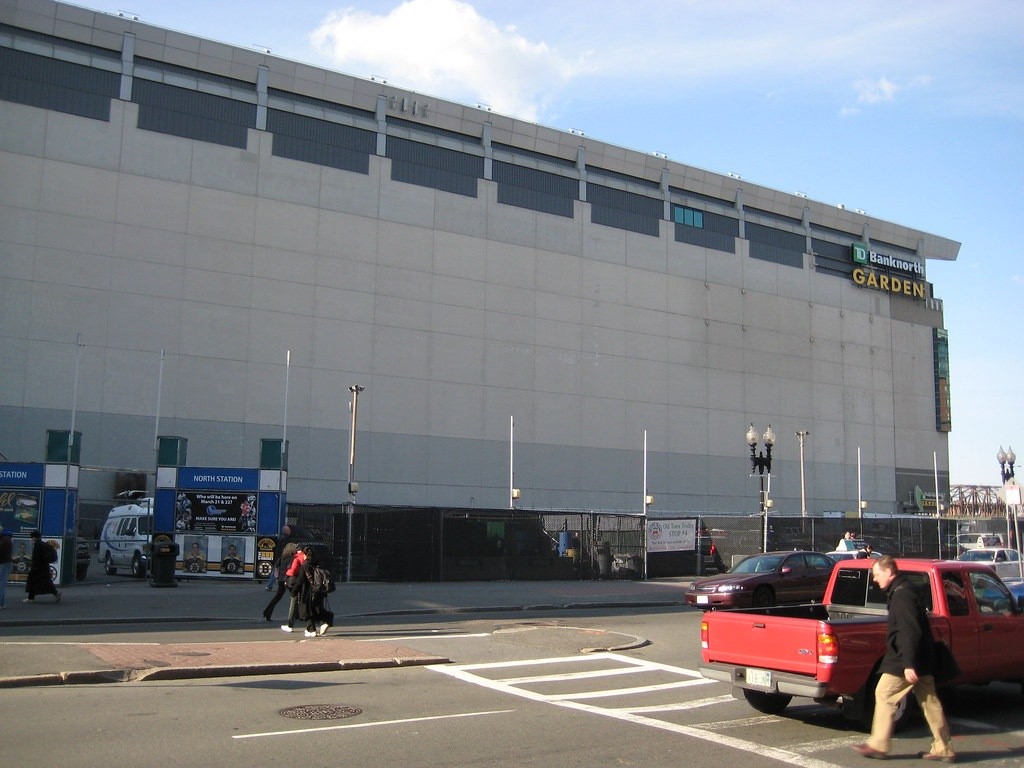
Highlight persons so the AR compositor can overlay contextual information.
[221,545,243,574]
[22,530,62,604]
[0,526,14,610]
[851,555,957,764]
[844,531,857,540]
[264,542,329,637]
[866,554,872,558]
[185,542,206,572]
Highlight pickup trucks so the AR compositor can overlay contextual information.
[698,558,1024,734]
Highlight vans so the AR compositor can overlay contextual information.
[98,497,154,577]
[945,532,1009,550]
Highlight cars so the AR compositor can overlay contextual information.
[75,536,91,581]
[814,539,883,565]
[699,530,823,572]
[954,536,1024,578]
[684,551,838,612]
[981,577,1023,606]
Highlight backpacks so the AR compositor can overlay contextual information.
[43,542,58,563]
[302,562,336,596]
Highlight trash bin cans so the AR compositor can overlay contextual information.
[152,543,179,586]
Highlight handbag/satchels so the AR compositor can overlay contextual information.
[322,598,335,627]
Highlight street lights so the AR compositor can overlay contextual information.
[998,446,1017,551]
[746,423,776,551]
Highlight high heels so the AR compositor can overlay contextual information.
[295,617,306,622]
[263,612,274,621]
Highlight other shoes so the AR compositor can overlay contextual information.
[264,587,274,592]
[0,605,7,610]
[281,625,293,633]
[319,623,329,635]
[917,751,957,763]
[304,630,317,637]
[54,590,62,603]
[849,743,888,759]
[22,598,35,604]
